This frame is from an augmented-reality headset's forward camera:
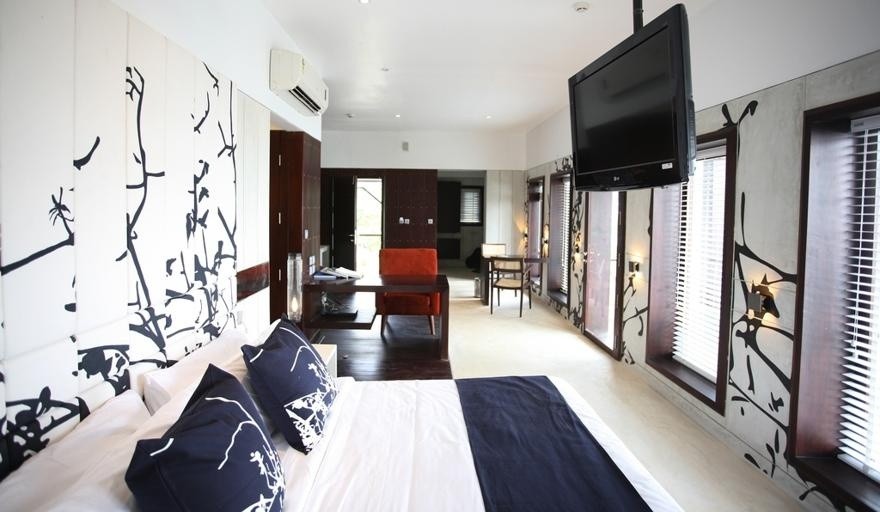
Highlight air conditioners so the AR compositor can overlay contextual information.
[270,47,329,117]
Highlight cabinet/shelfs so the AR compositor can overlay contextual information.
[271,130,321,277]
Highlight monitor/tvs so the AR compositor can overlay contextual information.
[567,3,695,192]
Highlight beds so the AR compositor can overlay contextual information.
[0,278,686,512]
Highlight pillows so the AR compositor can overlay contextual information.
[125,363,283,512]
[145,321,246,414]
[240,311,338,453]
[53,316,283,512]
[0,389,151,512]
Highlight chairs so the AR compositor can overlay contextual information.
[481,244,517,297]
[379,249,440,335]
[491,257,532,317]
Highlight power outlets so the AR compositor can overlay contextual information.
[405,219,410,224]
[428,218,434,224]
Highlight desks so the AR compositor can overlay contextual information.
[302,275,449,361]
